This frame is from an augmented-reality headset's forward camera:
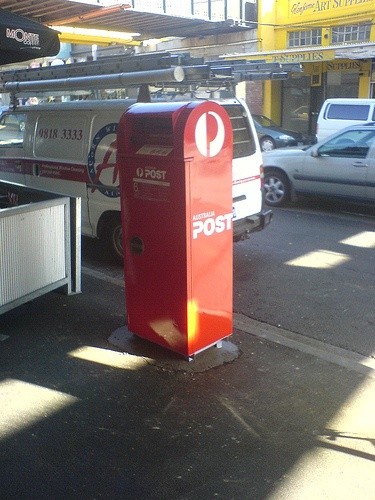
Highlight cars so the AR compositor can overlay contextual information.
[249,115,312,152]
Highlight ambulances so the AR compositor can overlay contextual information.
[0,98,271,268]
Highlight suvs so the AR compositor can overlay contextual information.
[262,121,375,207]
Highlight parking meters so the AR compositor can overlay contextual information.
[116,100,235,362]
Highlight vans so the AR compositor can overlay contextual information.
[314,98,375,144]
